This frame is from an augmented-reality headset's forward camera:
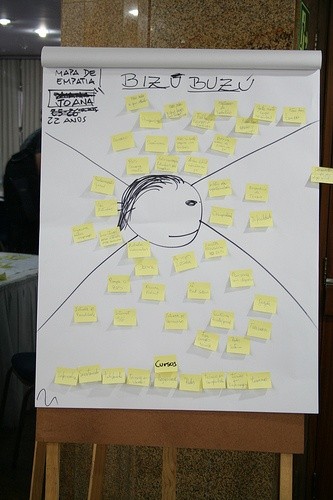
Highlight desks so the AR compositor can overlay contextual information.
[0,251,39,428]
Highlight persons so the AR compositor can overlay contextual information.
[3,126,42,254]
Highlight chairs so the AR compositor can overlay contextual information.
[0,352,35,474]
